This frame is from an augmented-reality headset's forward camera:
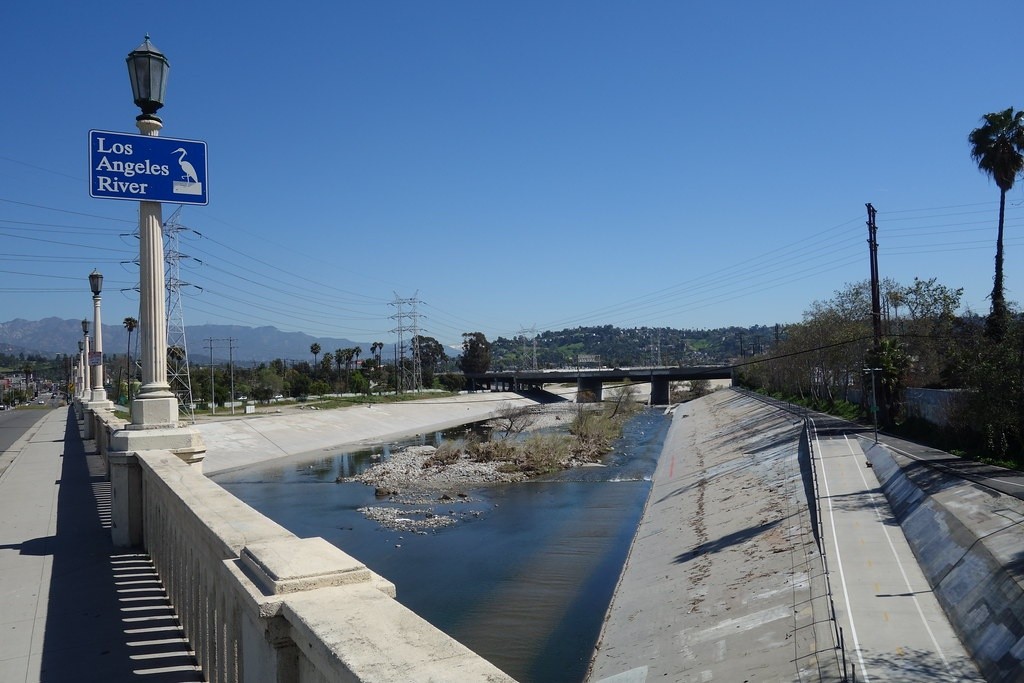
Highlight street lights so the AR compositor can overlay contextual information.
[125,33,187,431]
[69,265,108,401]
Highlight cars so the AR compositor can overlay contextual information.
[0,403,7,410]
[39,399,44,404]
